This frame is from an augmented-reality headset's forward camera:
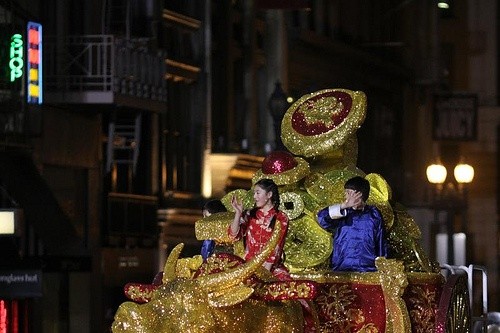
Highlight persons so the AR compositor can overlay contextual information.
[225,178,290,273]
[199,199,229,259]
[318,175,389,273]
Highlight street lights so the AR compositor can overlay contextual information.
[454,162,476,268]
[426,163,449,262]
